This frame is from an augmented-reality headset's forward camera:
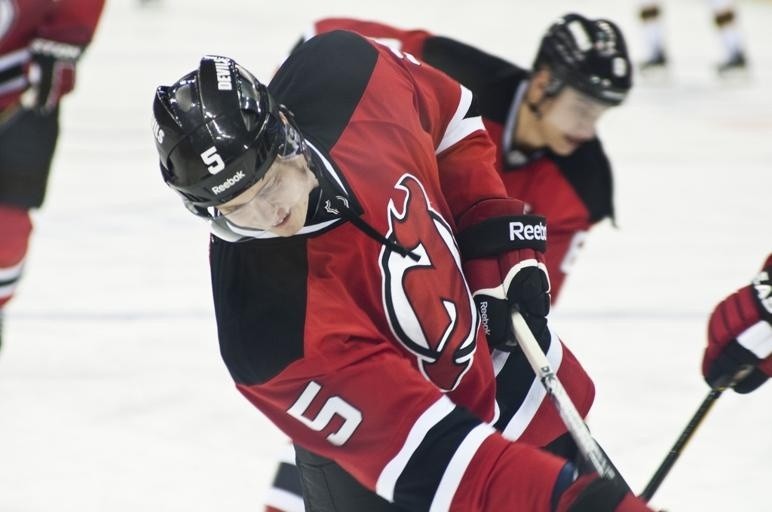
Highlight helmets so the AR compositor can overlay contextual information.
[152,56,307,242]
[533,14,632,132]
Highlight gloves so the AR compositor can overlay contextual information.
[556,474,657,512]
[452,198,550,351]
[20,38,81,113]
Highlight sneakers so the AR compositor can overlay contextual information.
[639,47,752,70]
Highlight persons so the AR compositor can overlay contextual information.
[260,13,631,510]
[0,1,108,316]
[151,29,647,512]
[637,2,743,70]
